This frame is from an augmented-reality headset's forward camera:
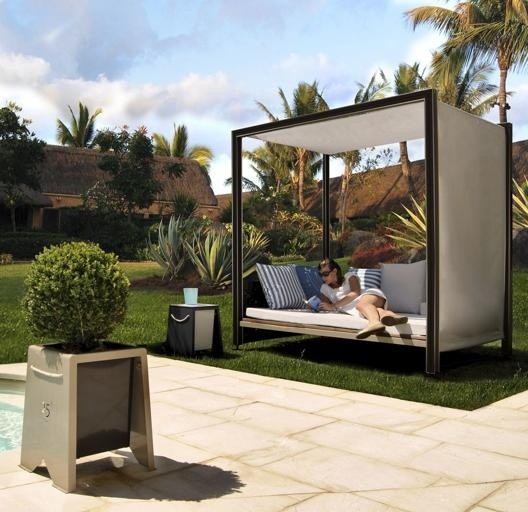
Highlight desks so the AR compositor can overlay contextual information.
[165,303,224,357]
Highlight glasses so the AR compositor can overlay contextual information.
[319,270,331,277]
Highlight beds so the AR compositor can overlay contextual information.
[233,306,512,375]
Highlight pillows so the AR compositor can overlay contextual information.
[252,260,425,314]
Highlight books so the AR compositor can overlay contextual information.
[301,296,322,312]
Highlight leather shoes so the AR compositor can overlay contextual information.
[381,315,409,326]
[356,322,385,339]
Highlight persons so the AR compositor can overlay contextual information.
[306,258,408,339]
